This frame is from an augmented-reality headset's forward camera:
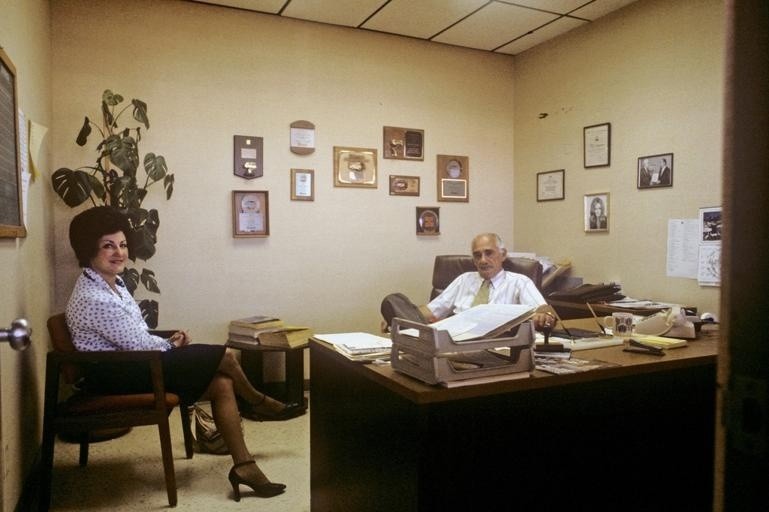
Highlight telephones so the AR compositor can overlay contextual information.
[636,306,695,340]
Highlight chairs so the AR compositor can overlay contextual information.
[429,253,542,304]
[37,315,194,507]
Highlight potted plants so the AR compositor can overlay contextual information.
[50,89,176,439]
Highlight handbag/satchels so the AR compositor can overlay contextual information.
[194,406,243,456]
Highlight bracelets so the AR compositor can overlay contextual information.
[545,311,555,320]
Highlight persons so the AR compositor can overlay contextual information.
[421,212,437,232]
[589,197,607,229]
[380,231,558,334]
[640,159,653,185]
[64,205,306,502]
[656,158,671,185]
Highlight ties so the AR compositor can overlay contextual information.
[471,279,492,307]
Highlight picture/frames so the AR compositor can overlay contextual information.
[232,190,269,239]
[636,153,674,190]
[583,191,611,235]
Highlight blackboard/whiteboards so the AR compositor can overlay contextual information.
[0,46,28,238]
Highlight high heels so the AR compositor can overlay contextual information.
[229,461,286,501]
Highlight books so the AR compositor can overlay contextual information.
[228,313,314,349]
[332,340,400,363]
[398,303,537,343]
[612,331,688,351]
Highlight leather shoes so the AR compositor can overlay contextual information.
[252,394,300,422]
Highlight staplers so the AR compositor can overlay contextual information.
[622,338,666,357]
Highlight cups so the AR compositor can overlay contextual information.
[605,310,635,339]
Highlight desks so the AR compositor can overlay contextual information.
[225,340,310,420]
[307,299,719,511]
